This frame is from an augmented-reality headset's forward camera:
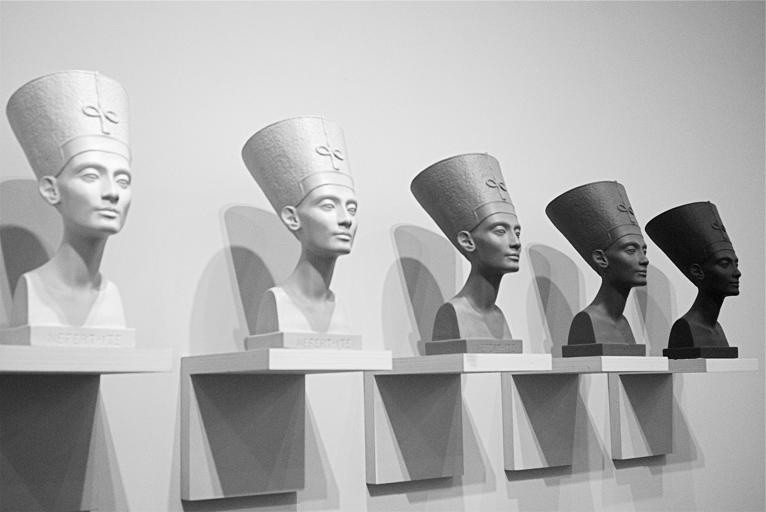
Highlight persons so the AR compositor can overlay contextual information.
[241,115,364,351]
[6,69,137,348]
[544,180,648,357]
[410,152,523,353]
[643,200,742,358]
